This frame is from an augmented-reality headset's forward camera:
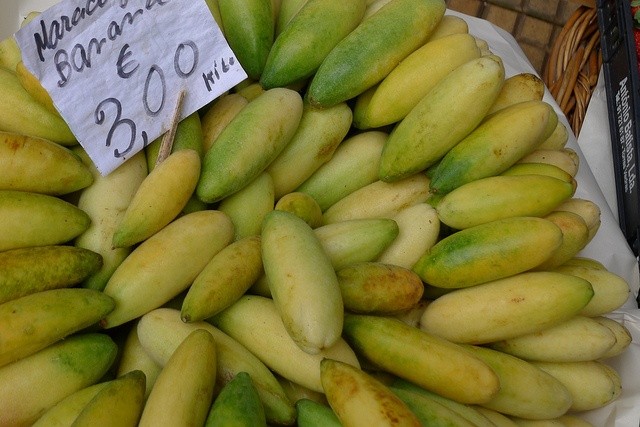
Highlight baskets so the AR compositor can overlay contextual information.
[598,2,639,307]
[544,2,604,141]
